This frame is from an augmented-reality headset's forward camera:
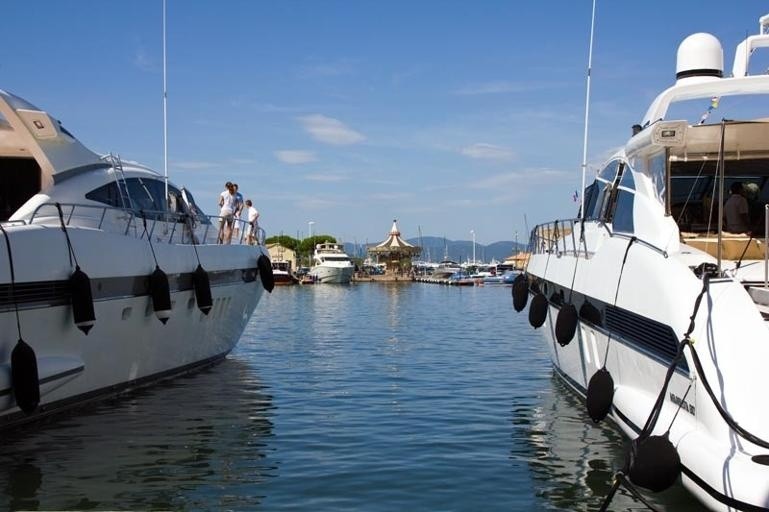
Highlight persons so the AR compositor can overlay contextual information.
[246,199,261,245]
[218,181,237,245]
[232,183,244,237]
[724,182,751,233]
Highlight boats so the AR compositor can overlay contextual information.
[514,1,769,511]
[270,220,520,285]
[1,1,273,448]
